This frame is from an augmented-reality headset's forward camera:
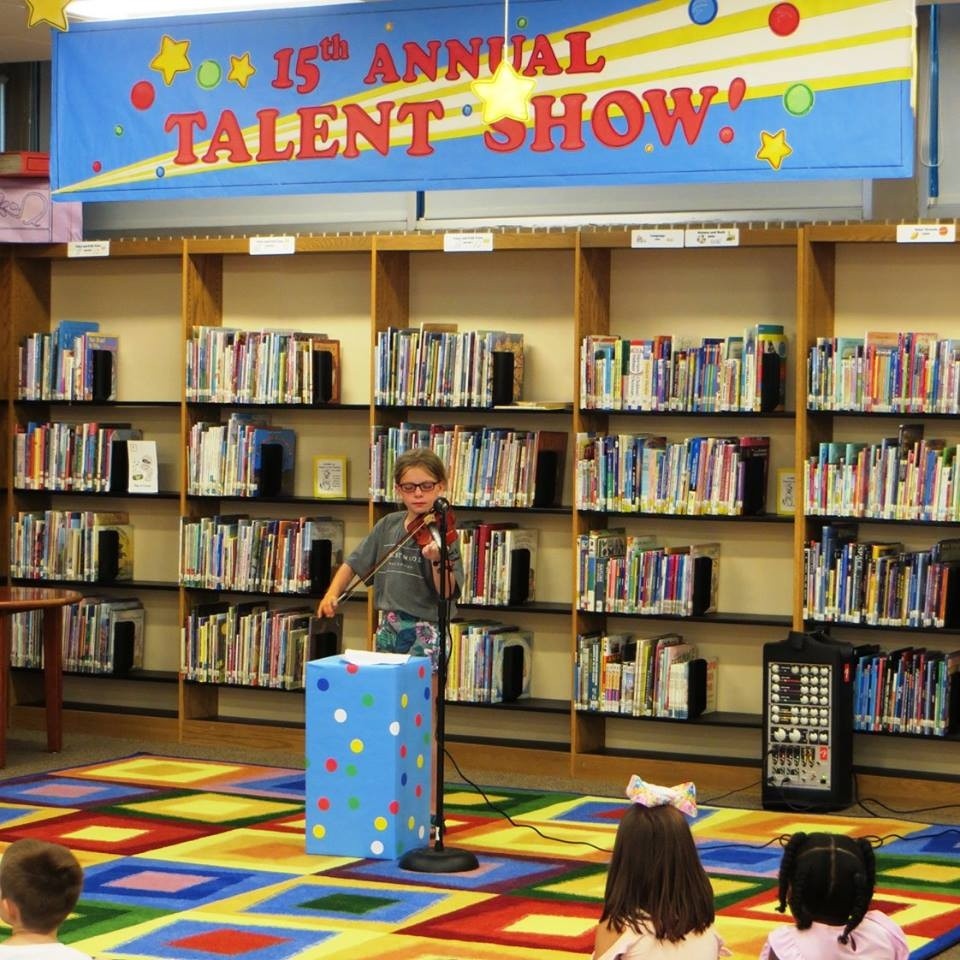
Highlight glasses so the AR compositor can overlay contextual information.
[398,481,441,492]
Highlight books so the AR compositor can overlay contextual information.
[187,412,296,498]
[376,323,523,407]
[575,432,770,516]
[11,609,44,669]
[580,324,785,411]
[10,510,134,581]
[372,422,568,508]
[803,524,960,738]
[777,468,795,515]
[185,325,340,404]
[13,422,158,492]
[807,331,960,413]
[804,424,960,521]
[178,516,345,593]
[313,454,347,498]
[18,320,118,401]
[180,601,343,690]
[444,621,717,719]
[62,596,146,672]
[453,521,721,615]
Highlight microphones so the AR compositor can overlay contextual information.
[434,499,449,513]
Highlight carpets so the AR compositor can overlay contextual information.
[0,750,960,960]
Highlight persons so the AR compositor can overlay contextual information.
[0,839,94,960]
[315,447,462,839]
[590,773,734,960]
[760,831,909,960]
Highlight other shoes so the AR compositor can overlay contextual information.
[430,815,446,835]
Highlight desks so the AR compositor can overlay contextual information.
[0,586,84,768]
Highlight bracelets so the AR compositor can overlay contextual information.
[432,560,440,566]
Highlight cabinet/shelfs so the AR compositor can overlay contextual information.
[0,223,960,788]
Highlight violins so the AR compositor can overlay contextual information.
[407,506,458,576]
